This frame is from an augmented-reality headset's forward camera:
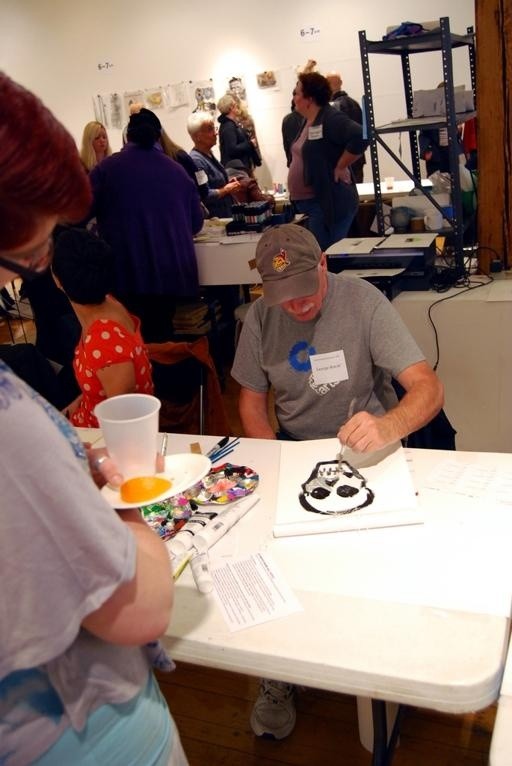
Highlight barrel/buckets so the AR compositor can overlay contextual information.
[382,205,410,234]
[423,208,442,232]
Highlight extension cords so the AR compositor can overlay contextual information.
[490,271,512,280]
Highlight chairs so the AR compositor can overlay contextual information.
[142,336,209,434]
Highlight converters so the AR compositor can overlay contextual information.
[491,259,502,272]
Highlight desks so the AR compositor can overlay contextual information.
[71,426,512,766]
[193,213,311,304]
[259,178,452,238]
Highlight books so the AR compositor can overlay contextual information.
[169,300,224,338]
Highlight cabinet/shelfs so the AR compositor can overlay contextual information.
[358,17,477,279]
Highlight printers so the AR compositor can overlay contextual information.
[324,233,437,296]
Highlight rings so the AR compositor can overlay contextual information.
[91,452,109,471]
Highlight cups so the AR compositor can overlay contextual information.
[381,204,454,233]
[92,393,162,488]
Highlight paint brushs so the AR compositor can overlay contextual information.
[209,435,241,464]
[338,396,358,469]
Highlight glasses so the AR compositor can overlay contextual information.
[0,238,51,285]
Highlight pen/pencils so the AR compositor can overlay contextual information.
[160,432,168,457]
[231,200,272,225]
[204,436,229,457]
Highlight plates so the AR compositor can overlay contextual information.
[101,452,212,513]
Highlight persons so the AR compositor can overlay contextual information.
[79,54,369,331]
[48,223,157,428]
[228,220,446,457]
[416,81,480,181]
[0,66,181,766]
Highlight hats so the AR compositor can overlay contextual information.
[255,223,323,306]
[224,159,249,173]
